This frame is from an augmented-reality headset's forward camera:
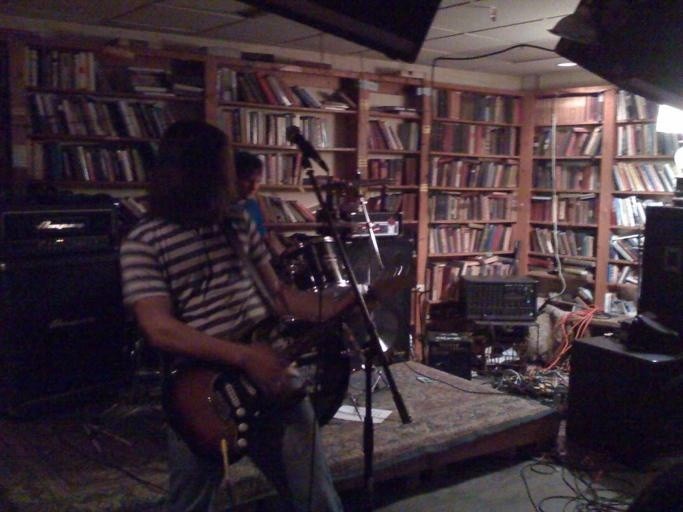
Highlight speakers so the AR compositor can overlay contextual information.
[0,209,124,418]
[347,237,413,363]
[637,208,683,312]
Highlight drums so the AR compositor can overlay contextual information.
[242,312,351,428]
[280,236,353,320]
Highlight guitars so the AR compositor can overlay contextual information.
[162,265,406,463]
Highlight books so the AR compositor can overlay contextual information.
[427,89,523,303]
[366,105,421,219]
[21,49,204,181]
[216,64,357,222]
[527,96,602,307]
[605,90,682,317]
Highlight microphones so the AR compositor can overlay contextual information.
[284,124,330,174]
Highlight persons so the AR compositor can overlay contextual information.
[118,118,346,511]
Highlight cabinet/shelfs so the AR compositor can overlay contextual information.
[0,27,683,418]
